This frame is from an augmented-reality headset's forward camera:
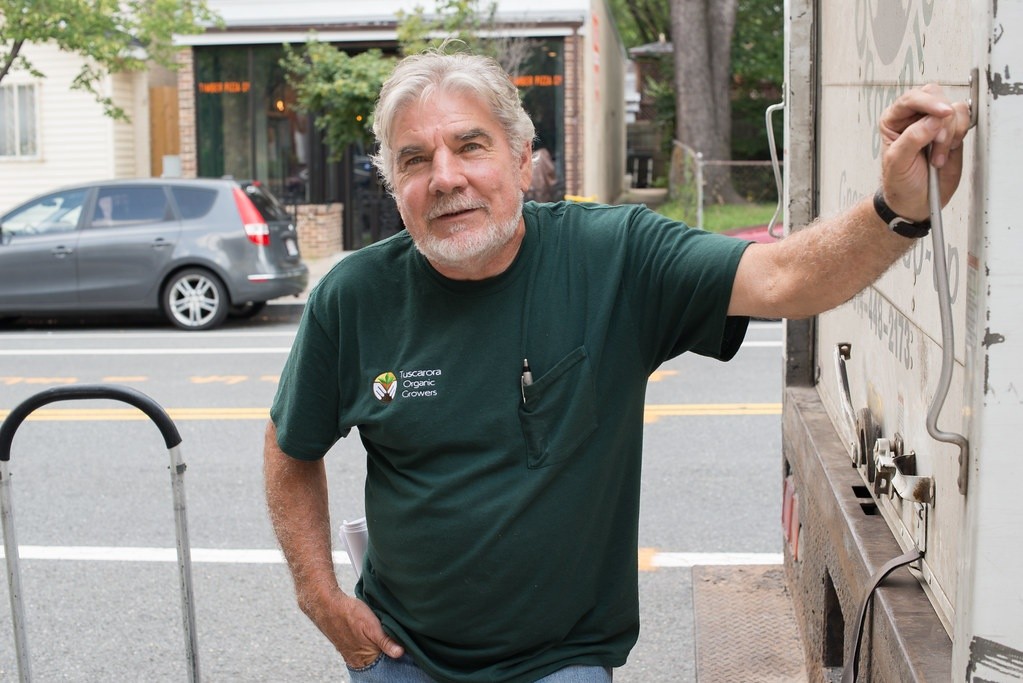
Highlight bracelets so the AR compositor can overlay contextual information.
[873,185,931,238]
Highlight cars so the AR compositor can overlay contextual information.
[0,174,309,331]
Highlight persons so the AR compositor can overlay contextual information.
[264,40,972,683]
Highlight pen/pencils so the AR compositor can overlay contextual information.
[521,358,534,404]
